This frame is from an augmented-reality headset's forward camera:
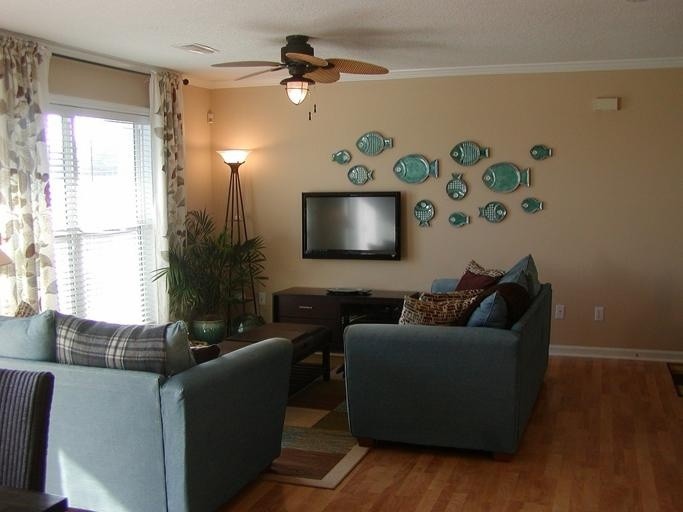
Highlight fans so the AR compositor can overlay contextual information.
[211,35,389,84]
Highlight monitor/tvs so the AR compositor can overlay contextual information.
[301,191,403,261]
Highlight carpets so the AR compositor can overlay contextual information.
[262,350,370,489]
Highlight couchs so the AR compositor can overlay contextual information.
[0,338,291,512]
[342,278,551,462]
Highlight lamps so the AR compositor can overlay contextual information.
[211,148,256,336]
[279,68,315,105]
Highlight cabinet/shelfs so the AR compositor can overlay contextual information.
[272,287,419,356]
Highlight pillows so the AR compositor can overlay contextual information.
[453,260,507,290]
[501,253,540,302]
[466,268,529,327]
[420,289,483,301]
[13,301,39,319]
[54,311,195,376]
[400,296,478,328]
[187,343,219,364]
[0,309,55,363]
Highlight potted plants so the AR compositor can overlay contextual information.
[154,210,263,346]
